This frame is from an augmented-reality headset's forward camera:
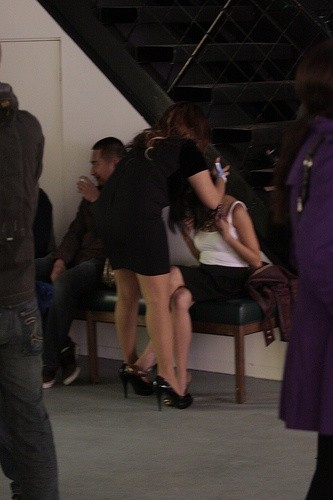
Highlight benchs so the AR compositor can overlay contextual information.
[72,291,280,403]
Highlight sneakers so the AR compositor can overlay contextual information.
[40,365,57,389]
[60,343,81,386]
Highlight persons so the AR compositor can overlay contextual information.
[32,100,262,413]
[0,81,59,500]
[274,41,333,500]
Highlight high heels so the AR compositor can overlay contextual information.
[118,363,193,409]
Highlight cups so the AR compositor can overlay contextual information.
[75,175,99,187]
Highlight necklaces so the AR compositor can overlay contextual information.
[205,199,222,231]
